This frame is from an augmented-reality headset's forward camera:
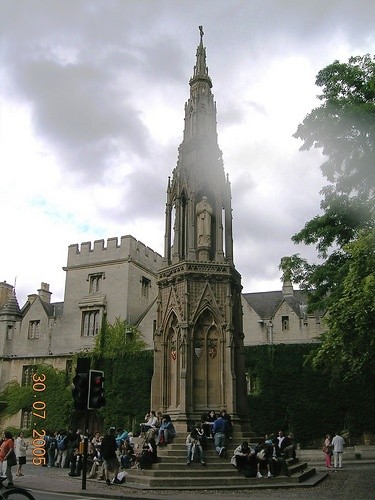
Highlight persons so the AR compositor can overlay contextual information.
[186,409,233,465]
[230,431,296,479]
[324,434,334,468]
[195,195,213,246]
[0,430,14,489]
[331,432,345,468]
[41,426,135,486]
[134,411,176,473]
[15,431,29,477]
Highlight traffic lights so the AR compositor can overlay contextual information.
[71,371,88,411]
[87,370,106,411]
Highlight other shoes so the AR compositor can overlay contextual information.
[199,460,206,464]
[105,479,110,484]
[16,473,24,477]
[216,447,220,453]
[6,482,14,488]
[87,475,95,479]
[218,447,226,456]
[163,442,169,446]
[257,472,263,478]
[48,465,53,468]
[186,460,190,464]
[267,473,272,477]
[97,477,104,481]
[229,436,233,439]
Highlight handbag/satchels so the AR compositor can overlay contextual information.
[323,446,329,453]
[0,460,7,478]
[160,435,164,446]
[331,451,333,456]
[7,454,18,467]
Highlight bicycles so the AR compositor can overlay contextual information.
[0,475,35,500]
